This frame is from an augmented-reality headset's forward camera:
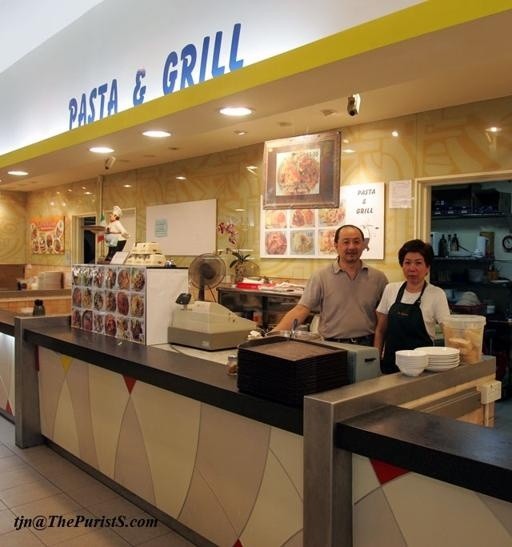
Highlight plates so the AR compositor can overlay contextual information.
[416,347,461,373]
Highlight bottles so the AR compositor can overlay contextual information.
[438,234,459,256]
[32,300,45,315]
[225,355,239,377]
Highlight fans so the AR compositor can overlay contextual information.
[187,253,227,301]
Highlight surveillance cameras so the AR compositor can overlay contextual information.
[105,157,116,169]
[347,94,360,116]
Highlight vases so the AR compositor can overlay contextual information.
[233,264,245,283]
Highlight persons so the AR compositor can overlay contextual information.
[272,224,452,375]
[89,206,130,264]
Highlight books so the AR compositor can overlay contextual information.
[259,282,305,294]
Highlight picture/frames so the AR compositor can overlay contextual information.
[261,130,342,210]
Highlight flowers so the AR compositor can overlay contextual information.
[217,221,262,268]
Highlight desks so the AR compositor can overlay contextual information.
[216,281,324,333]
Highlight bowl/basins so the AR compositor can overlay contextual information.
[395,350,428,377]
[265,330,323,340]
